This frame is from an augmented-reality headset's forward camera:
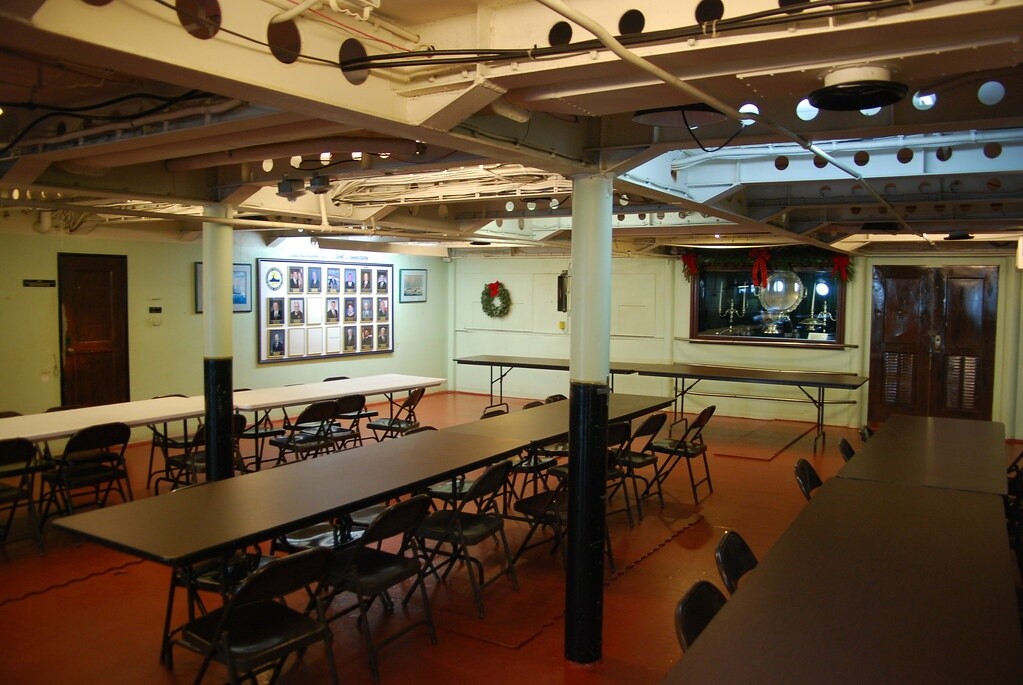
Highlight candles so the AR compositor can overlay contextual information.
[718,280,724,311]
[742,281,747,311]
[811,282,817,314]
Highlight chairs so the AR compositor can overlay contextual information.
[0,373,877,685]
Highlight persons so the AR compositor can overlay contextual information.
[270,301,282,320]
[345,273,355,289]
[378,301,388,316]
[378,272,386,289]
[290,300,303,319]
[345,301,356,317]
[362,328,371,345]
[361,271,370,288]
[272,334,283,355]
[290,269,300,288]
[327,301,338,318]
[378,326,387,344]
[310,270,319,288]
[345,326,354,346]
[362,299,373,318]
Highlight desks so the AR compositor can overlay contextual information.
[453,355,869,456]
[0,373,1023,685]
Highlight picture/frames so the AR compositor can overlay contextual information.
[399,269,427,303]
[344,268,356,294]
[343,297,357,325]
[376,269,389,295]
[360,297,373,323]
[287,266,304,294]
[326,267,340,295]
[266,297,285,327]
[266,329,285,358]
[232,263,253,313]
[377,324,390,350]
[287,297,305,326]
[377,297,389,323]
[307,266,322,294]
[326,297,340,325]
[360,325,374,351]
[360,268,373,295]
[343,326,357,352]
